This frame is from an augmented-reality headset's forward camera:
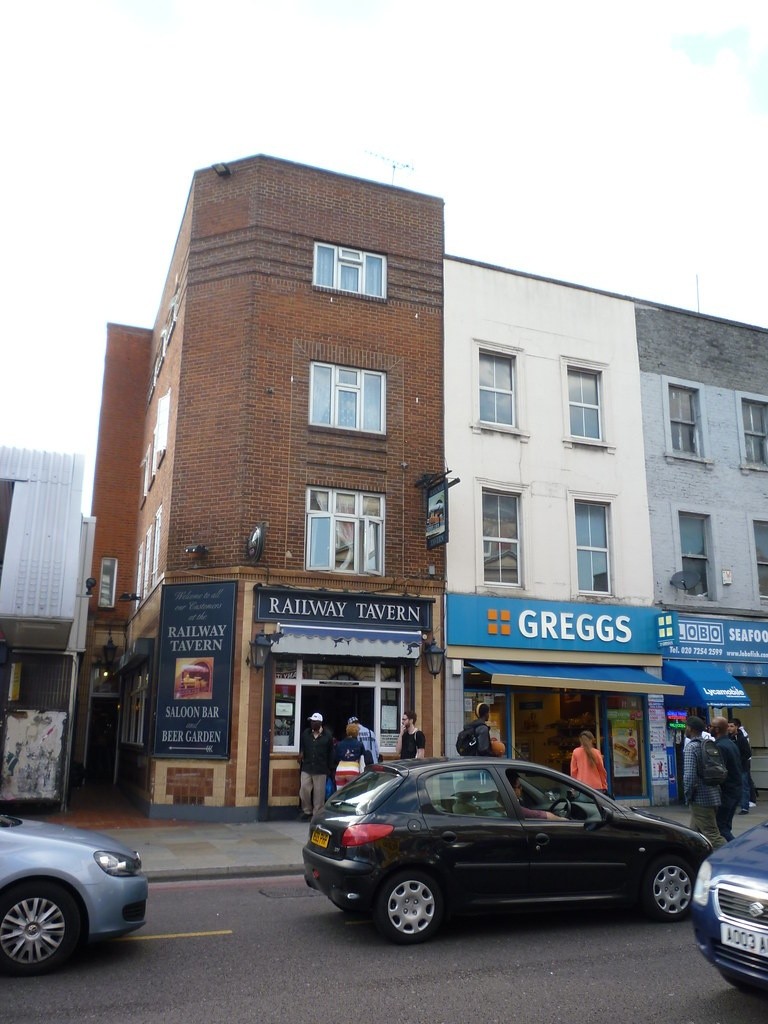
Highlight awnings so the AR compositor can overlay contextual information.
[469,659,685,699]
[662,660,753,707]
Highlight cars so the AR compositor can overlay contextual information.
[0,814,150,978]
[302,756,713,946]
[690,817,768,991]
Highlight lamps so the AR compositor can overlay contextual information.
[104,631,118,673]
[86,578,97,595]
[213,160,231,178]
[247,632,287,668]
[422,640,445,679]
[116,592,142,603]
[185,545,207,554]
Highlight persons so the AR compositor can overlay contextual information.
[299,711,426,820]
[506,772,570,820]
[456,703,505,756]
[682,713,757,853]
[570,730,608,795]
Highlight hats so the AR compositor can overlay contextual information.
[348,717,358,724]
[682,716,704,731]
[308,712,323,722]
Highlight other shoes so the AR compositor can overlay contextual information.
[739,810,749,815]
[749,802,756,807]
[301,814,313,822]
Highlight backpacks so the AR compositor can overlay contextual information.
[456,723,485,756]
[691,739,728,786]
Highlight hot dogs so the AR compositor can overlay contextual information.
[614,742,637,762]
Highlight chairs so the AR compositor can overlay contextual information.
[476,783,507,815]
[451,781,475,817]
